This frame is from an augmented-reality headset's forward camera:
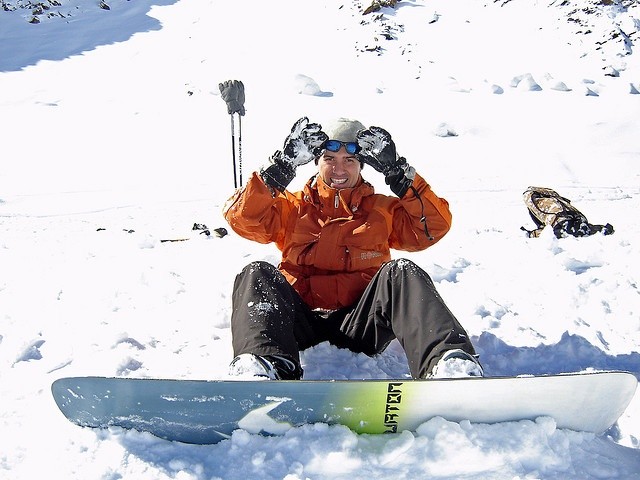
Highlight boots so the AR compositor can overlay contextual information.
[230,351,304,381]
[429,349,485,378]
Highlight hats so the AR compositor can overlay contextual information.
[321,118,365,143]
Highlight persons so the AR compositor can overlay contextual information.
[222,117,485,379]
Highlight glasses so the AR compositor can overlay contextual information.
[322,139,359,154]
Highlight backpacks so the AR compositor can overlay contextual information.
[520,186,615,239]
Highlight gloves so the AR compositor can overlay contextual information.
[219,80,241,114]
[232,80,245,115]
[260,117,329,193]
[355,126,416,198]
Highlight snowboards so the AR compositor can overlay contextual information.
[49,370,638,445]
